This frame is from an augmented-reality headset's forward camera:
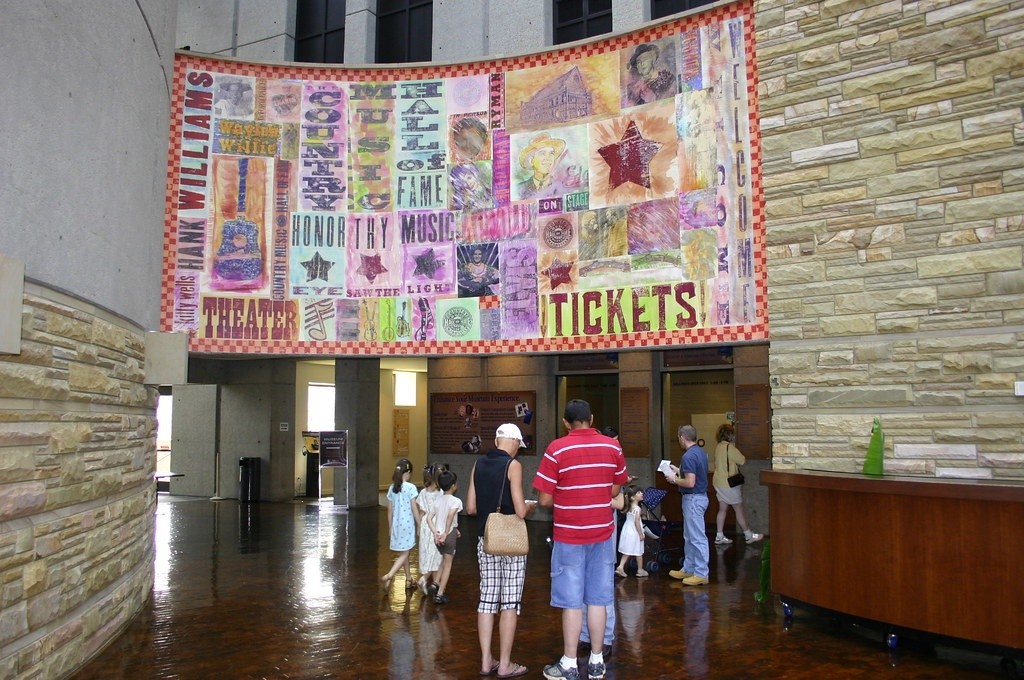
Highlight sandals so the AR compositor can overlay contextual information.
[636,569,649,577]
[745,533,764,544]
[714,536,733,544]
[615,566,627,578]
[406,578,417,588]
[430,582,440,593]
[381,574,393,593]
[435,595,448,603]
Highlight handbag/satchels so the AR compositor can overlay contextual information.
[482,513,529,556]
[728,474,746,488]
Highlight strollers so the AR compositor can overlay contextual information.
[616,486,682,573]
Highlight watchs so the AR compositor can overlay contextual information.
[672,479,675,484]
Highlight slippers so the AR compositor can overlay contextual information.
[479,661,500,675]
[496,663,529,678]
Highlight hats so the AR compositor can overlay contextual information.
[496,423,527,448]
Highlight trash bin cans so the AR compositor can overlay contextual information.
[239,457,262,504]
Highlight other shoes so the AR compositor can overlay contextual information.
[601,643,611,655]
[578,641,592,651]
[418,577,428,595]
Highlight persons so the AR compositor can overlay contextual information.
[665,425,709,585]
[531,399,628,680]
[416,462,463,604]
[381,459,421,596]
[577,486,624,662]
[615,485,649,577]
[713,424,764,544]
[466,423,537,678]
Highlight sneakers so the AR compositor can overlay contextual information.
[682,574,709,585]
[542,663,581,680]
[586,660,607,680]
[669,569,690,579]
